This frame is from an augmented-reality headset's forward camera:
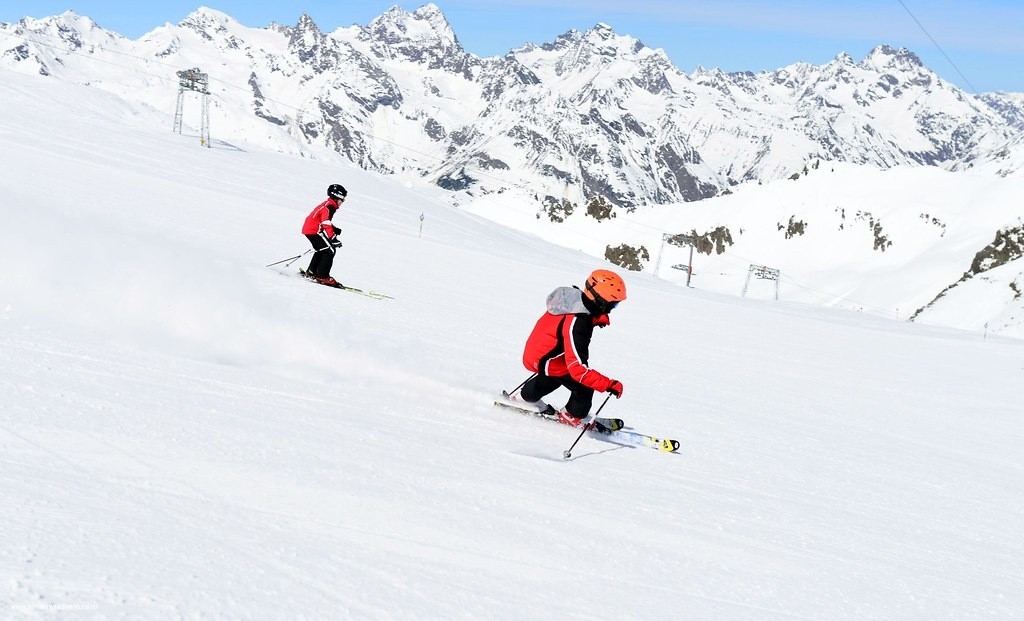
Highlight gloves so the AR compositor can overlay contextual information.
[334,227,341,235]
[332,236,342,248]
[592,316,610,328]
[606,379,623,399]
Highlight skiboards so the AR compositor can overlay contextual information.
[493,391,681,454]
[298,268,397,301]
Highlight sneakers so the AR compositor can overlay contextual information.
[305,269,315,277]
[511,390,546,412]
[314,275,336,286]
[559,407,594,430]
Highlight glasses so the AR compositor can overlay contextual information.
[606,302,621,309]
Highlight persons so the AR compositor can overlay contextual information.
[301,184,347,285]
[512,269,627,428]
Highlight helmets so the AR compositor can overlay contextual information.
[327,184,347,200]
[584,270,626,304]
